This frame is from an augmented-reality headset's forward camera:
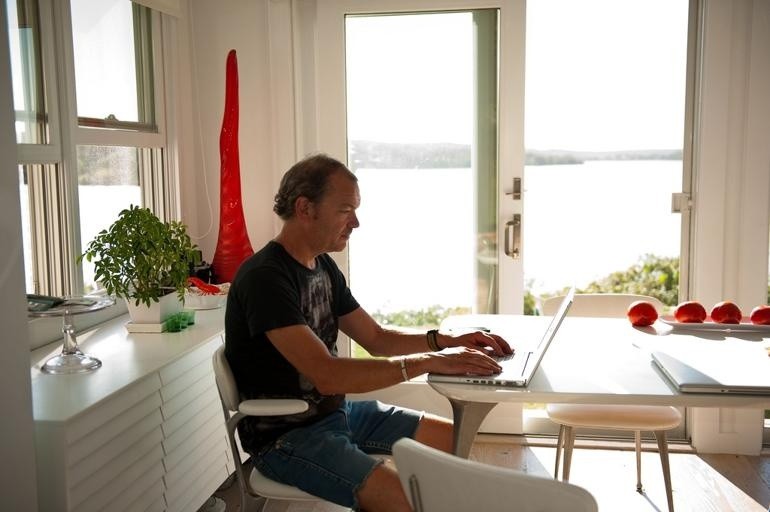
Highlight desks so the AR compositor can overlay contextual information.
[423,308,769,460]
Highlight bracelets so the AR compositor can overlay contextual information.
[425,328,445,353]
[398,353,410,383]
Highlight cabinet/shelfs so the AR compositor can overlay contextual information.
[29,286,251,512]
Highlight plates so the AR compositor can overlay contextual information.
[659,312,770,334]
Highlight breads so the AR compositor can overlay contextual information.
[628,303,658,327]
[751,305,770,323]
[675,302,706,323]
[710,302,743,323]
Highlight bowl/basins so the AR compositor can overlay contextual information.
[183,291,225,308]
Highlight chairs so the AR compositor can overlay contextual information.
[541,290,682,511]
[206,341,379,512]
[390,438,601,511]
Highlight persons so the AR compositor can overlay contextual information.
[224,151,516,511]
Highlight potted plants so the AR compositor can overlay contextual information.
[82,208,203,334]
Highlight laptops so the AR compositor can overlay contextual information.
[650,347,769,394]
[426,290,577,388]
